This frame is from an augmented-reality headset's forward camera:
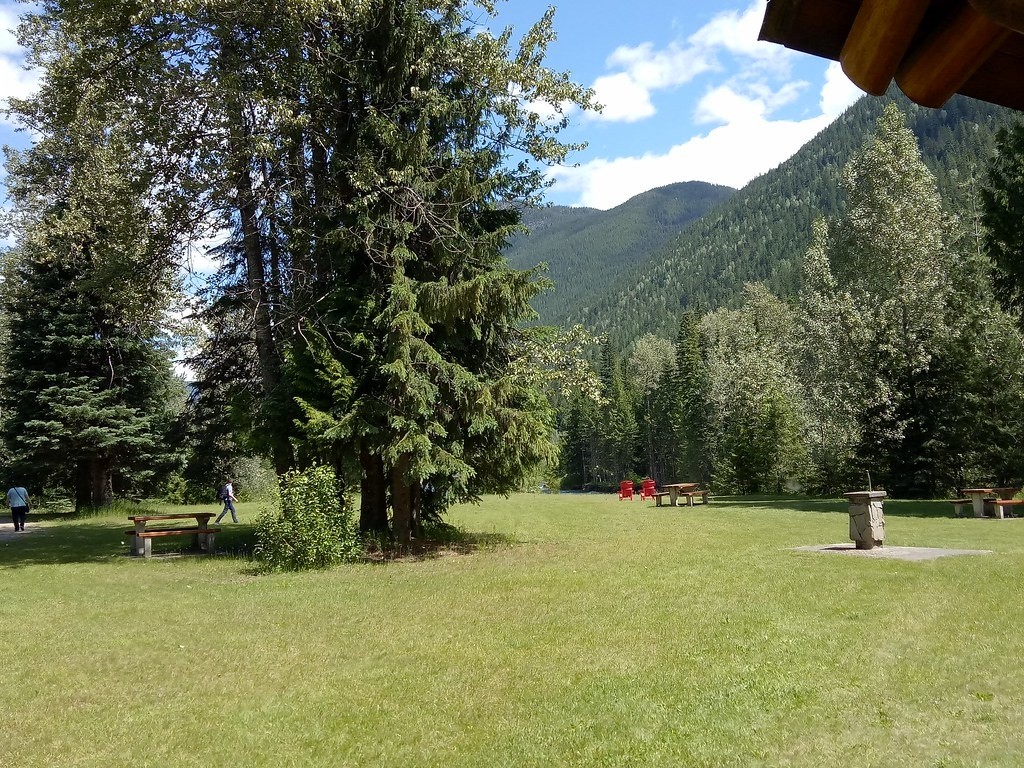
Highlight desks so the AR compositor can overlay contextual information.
[961,487,1021,518]
[128,513,216,556]
[662,483,700,506]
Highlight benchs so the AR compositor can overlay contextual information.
[682,490,710,507]
[988,500,1024,519]
[950,499,1011,517]
[125,526,221,558]
[652,492,680,507]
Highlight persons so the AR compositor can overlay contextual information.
[215,479,239,525]
[6,481,32,532]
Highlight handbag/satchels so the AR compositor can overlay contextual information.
[24,502,30,513]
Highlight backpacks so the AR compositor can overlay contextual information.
[219,485,231,506]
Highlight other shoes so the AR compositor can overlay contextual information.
[20,525,25,531]
[15,527,19,532]
[214,522,220,525]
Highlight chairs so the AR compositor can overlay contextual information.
[617,481,634,501]
[640,480,656,501]
